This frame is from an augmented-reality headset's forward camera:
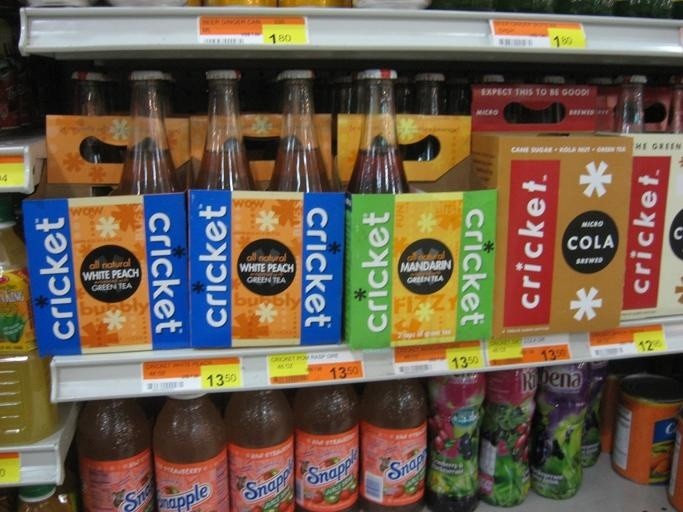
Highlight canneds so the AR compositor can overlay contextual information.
[669,411,683,510]
[610,373,680,485]
[601,372,627,456]
[0,4,50,130]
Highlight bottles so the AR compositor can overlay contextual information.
[480,369,537,505]
[0,208,74,511]
[224,391,294,512]
[359,380,427,511]
[293,387,360,512]
[153,392,227,511]
[76,399,155,512]
[614,73,649,133]
[266,68,331,193]
[195,71,255,191]
[424,375,488,512]
[111,69,177,194]
[582,362,608,466]
[342,67,409,193]
[533,362,591,498]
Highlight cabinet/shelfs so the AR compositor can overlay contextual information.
[0,8,683,512]
